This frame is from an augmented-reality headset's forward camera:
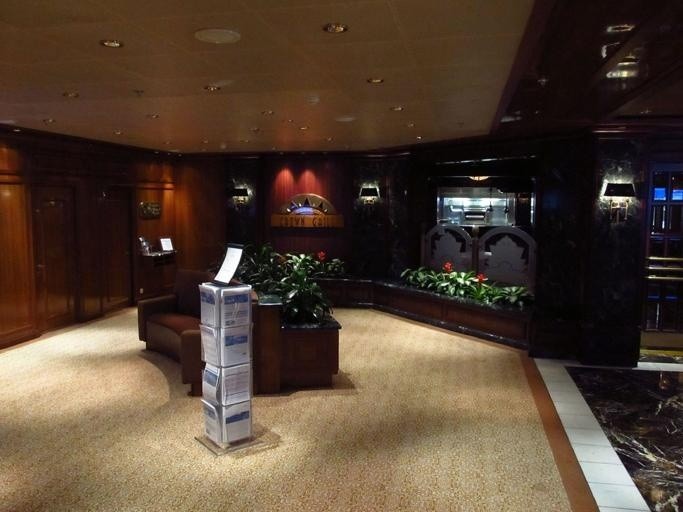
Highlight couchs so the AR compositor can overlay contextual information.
[138,269,258,395]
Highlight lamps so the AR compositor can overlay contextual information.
[232,190,248,205]
[361,187,380,204]
[602,181,636,226]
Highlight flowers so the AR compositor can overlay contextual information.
[311,252,345,276]
[398,261,529,303]
